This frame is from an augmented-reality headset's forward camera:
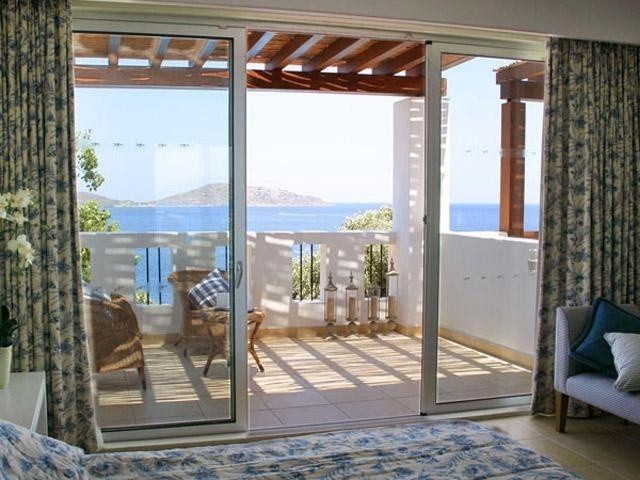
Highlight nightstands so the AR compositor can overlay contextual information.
[0,371,48,435]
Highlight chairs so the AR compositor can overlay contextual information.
[554,302,640,433]
[168,268,228,357]
[81,279,147,393]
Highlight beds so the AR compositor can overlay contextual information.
[0,420,591,480]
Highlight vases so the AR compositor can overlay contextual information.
[0,344,12,386]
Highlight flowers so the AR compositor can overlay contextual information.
[0,187,41,348]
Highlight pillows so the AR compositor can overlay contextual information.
[186,268,230,309]
[571,297,640,393]
[91,286,112,303]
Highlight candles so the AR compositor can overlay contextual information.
[327,297,394,321]
